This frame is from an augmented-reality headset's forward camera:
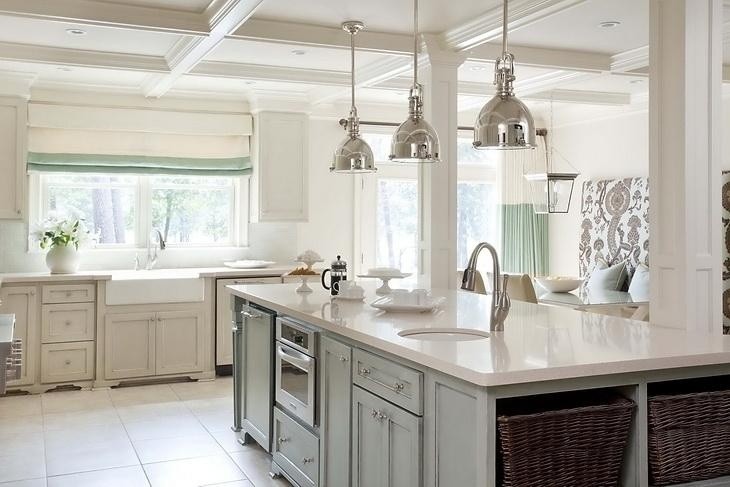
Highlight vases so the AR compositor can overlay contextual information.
[47,246,82,274]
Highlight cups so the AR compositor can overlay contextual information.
[322,254,347,296]
[392,289,427,305]
[322,298,343,325]
[334,280,363,297]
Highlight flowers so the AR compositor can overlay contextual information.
[28,212,102,251]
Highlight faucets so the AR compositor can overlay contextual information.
[459,241,513,333]
[145,227,166,271]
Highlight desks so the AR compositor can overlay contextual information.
[0,312,16,394]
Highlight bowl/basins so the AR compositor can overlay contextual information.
[535,275,584,292]
[540,292,581,303]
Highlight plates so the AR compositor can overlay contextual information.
[373,296,447,314]
[225,260,277,269]
[331,295,365,302]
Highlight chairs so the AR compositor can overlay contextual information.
[485,271,538,304]
[457,268,486,295]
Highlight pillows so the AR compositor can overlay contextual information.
[628,264,650,296]
[587,258,628,291]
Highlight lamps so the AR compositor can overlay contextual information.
[523,92,580,215]
[387,0,442,165]
[472,0,539,152]
[329,22,378,175]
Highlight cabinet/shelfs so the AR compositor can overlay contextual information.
[39,284,96,390]
[316,333,351,487]
[249,112,310,225]
[0,285,36,392]
[102,310,208,384]
[0,96,28,221]
[352,348,429,487]
[213,277,284,367]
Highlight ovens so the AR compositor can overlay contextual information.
[274,340,319,425]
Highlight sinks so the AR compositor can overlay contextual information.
[103,269,204,307]
[397,325,492,342]
